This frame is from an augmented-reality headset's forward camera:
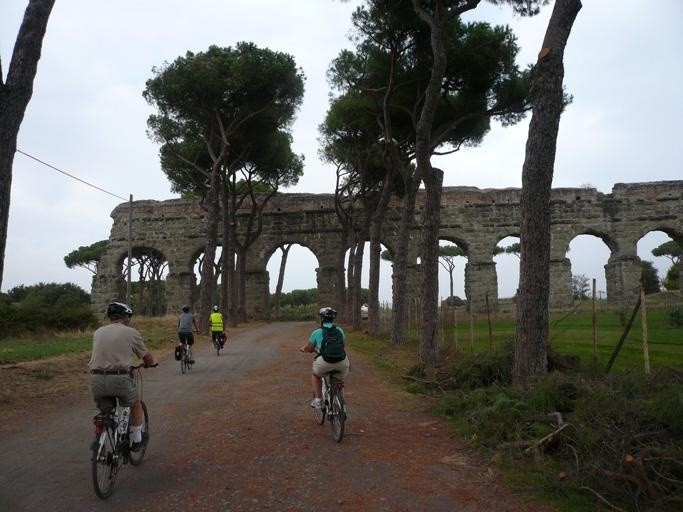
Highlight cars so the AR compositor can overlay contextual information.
[361,302,369,319]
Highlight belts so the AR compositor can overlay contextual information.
[94,369,129,375]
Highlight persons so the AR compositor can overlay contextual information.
[300,306,350,423]
[208,305,224,349]
[87,302,158,452]
[177,304,199,364]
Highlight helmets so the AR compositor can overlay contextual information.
[213,305,219,310]
[319,307,338,318]
[183,304,190,311]
[107,303,133,317]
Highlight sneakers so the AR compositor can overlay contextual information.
[132,432,148,451]
[311,399,325,409]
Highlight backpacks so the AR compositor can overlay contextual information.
[320,324,346,363]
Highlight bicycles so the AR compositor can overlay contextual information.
[179,329,200,374]
[301,348,347,443]
[207,324,224,356]
[90,362,158,500]
